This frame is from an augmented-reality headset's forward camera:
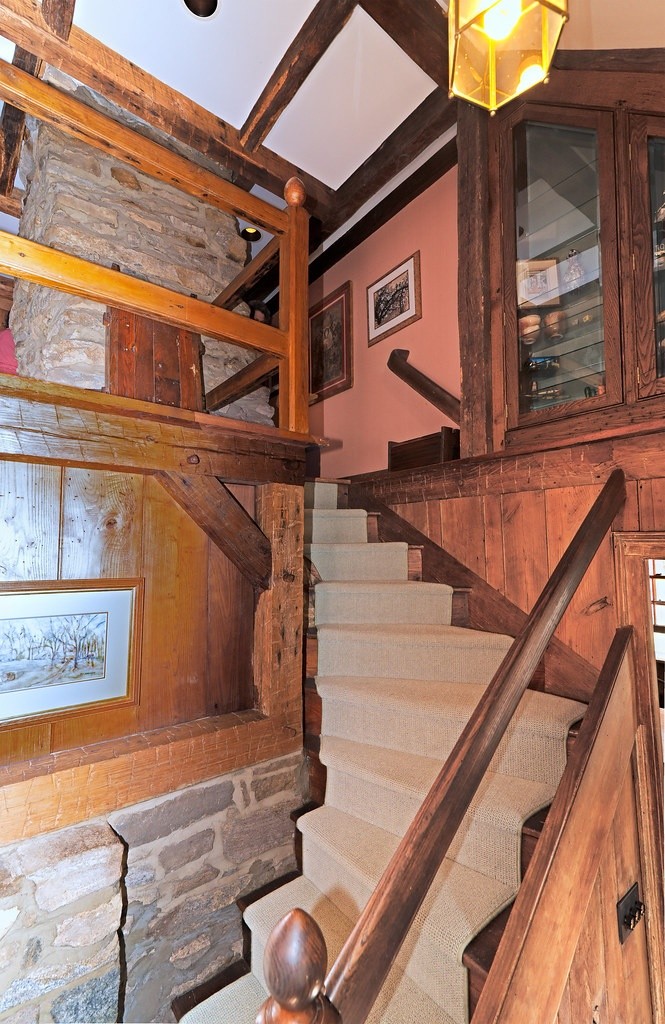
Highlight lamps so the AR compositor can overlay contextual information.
[447,0,568,117]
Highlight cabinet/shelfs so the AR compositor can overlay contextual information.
[457,49,664,459]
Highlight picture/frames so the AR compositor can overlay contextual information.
[366,249,422,348]
[516,257,561,309]
[308,280,353,407]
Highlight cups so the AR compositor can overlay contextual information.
[517,314,541,345]
[543,310,567,343]
